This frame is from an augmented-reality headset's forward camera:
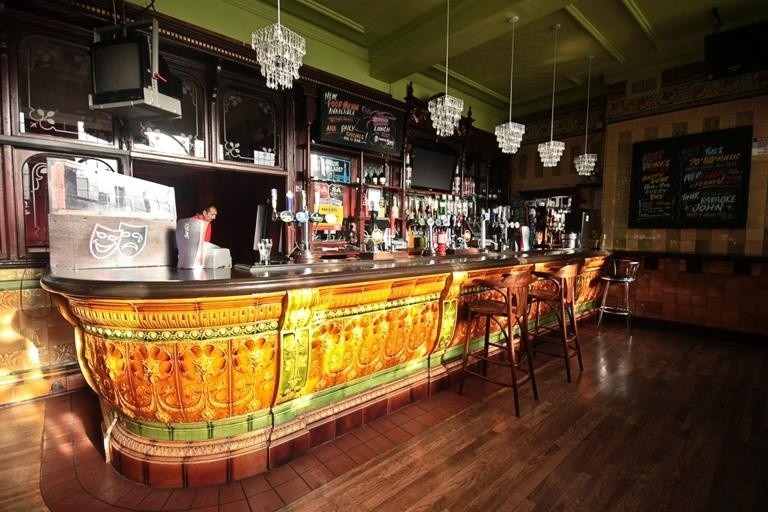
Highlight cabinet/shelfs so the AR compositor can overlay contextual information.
[296,120,508,259]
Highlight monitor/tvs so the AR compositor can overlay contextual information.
[89,34,183,105]
[411,139,459,194]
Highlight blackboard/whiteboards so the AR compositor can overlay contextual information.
[627,124,753,229]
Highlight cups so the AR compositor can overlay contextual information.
[257,238,274,265]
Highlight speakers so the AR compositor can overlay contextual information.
[704,20,768,81]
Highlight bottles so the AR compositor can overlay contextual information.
[405,152,413,189]
[451,164,461,195]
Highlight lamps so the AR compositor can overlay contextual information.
[537,24,567,168]
[250,1,307,91]
[573,55,598,177]
[428,0,464,138]
[494,15,526,155]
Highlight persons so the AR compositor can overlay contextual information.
[190,204,218,243]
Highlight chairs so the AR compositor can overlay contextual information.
[597,259,640,332]
[459,264,584,417]
